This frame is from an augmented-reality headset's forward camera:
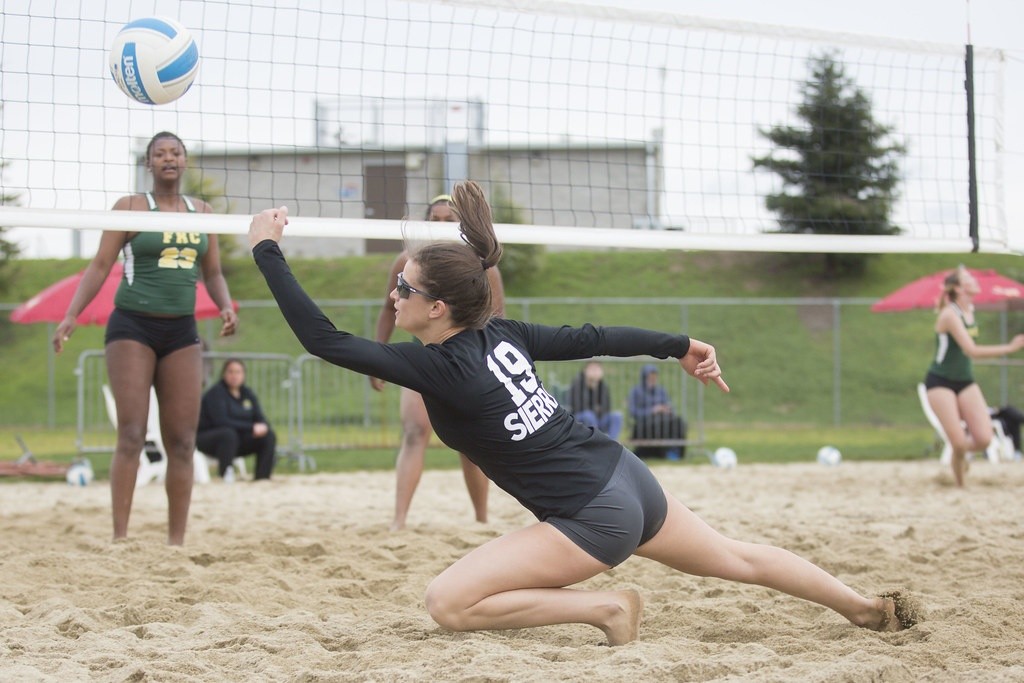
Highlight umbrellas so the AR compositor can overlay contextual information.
[871,258,1024,452]
[7,258,241,479]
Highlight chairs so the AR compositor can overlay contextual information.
[915,383,1016,467]
[100,384,210,485]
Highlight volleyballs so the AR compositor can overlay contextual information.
[817,444,842,465]
[106,16,200,107]
[64,461,94,487]
[712,445,739,466]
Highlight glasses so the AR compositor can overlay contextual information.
[397,272,457,305]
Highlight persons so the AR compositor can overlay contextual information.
[53,131,239,548]
[367,195,504,532]
[925,268,1024,487]
[628,363,689,463]
[246,177,903,648]
[562,359,622,443]
[196,357,277,482]
